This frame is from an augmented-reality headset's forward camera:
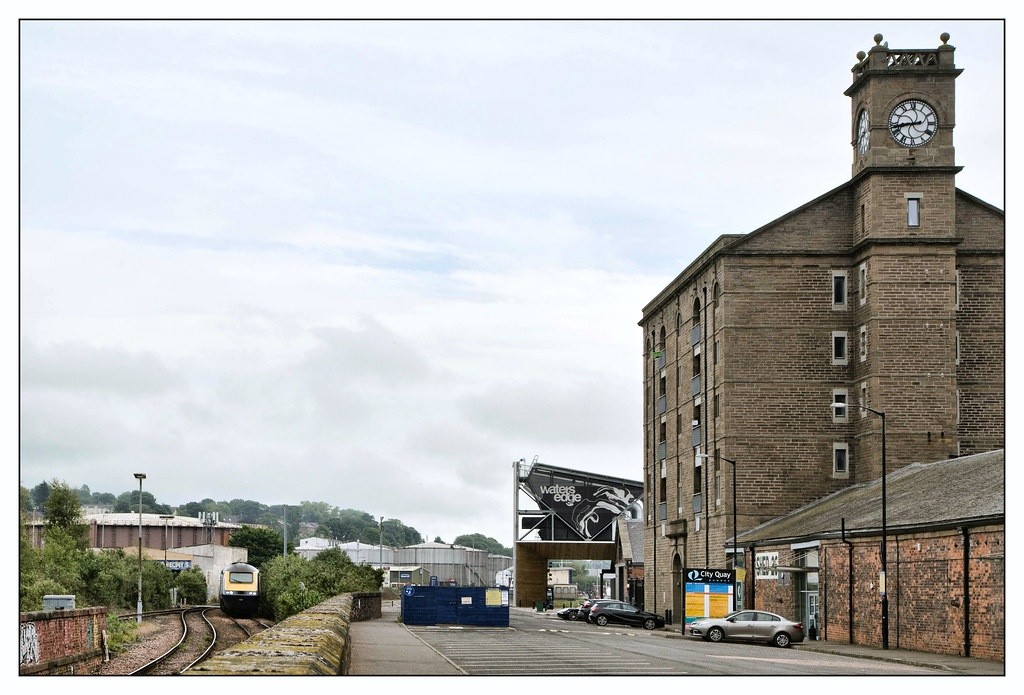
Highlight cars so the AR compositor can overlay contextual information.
[688,611,806,649]
[557,607,579,621]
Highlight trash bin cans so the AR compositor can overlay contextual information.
[536,601,545,612]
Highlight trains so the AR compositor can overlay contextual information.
[218,561,261,617]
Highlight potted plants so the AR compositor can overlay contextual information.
[816,627,820,641]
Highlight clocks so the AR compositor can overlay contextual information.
[856,107,870,156]
[888,99,939,147]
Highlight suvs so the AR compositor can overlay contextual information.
[577,599,621,624]
[588,601,665,631]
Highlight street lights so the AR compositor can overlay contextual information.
[829,402,890,650]
[132,472,147,623]
[695,453,739,569]
[159,515,175,566]
